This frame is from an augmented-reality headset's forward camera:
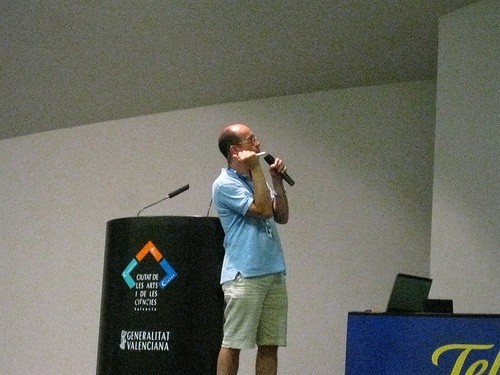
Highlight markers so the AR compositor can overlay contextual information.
[256,152,266,157]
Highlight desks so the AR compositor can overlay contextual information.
[345,311,500,375]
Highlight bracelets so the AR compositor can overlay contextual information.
[273,187,287,197]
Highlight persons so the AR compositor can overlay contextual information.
[212,124,289,375]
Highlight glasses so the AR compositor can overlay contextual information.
[226,136,257,151]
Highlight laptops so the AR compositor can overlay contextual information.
[364,273,432,315]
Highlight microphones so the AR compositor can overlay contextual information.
[264,154,295,187]
[137,184,189,216]
